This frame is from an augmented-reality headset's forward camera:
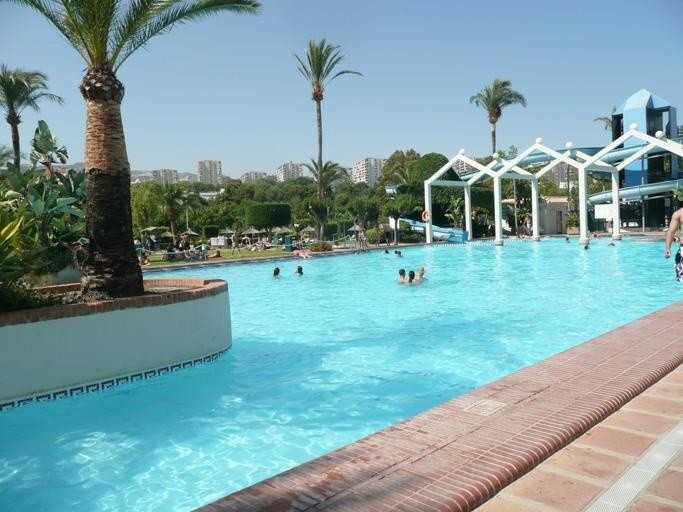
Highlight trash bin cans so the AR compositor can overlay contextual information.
[285,235,297,252]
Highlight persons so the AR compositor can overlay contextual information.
[405,271,417,285]
[665,207,683,282]
[519,232,524,239]
[524,214,532,236]
[273,268,280,277]
[397,269,408,283]
[232,232,241,256]
[138,233,220,266]
[375,224,402,257]
[416,267,424,281]
[296,265,303,275]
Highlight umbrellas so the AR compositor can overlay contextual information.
[140,226,199,239]
[349,225,362,231]
[222,225,315,239]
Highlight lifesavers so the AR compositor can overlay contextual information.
[422,211,430,222]
